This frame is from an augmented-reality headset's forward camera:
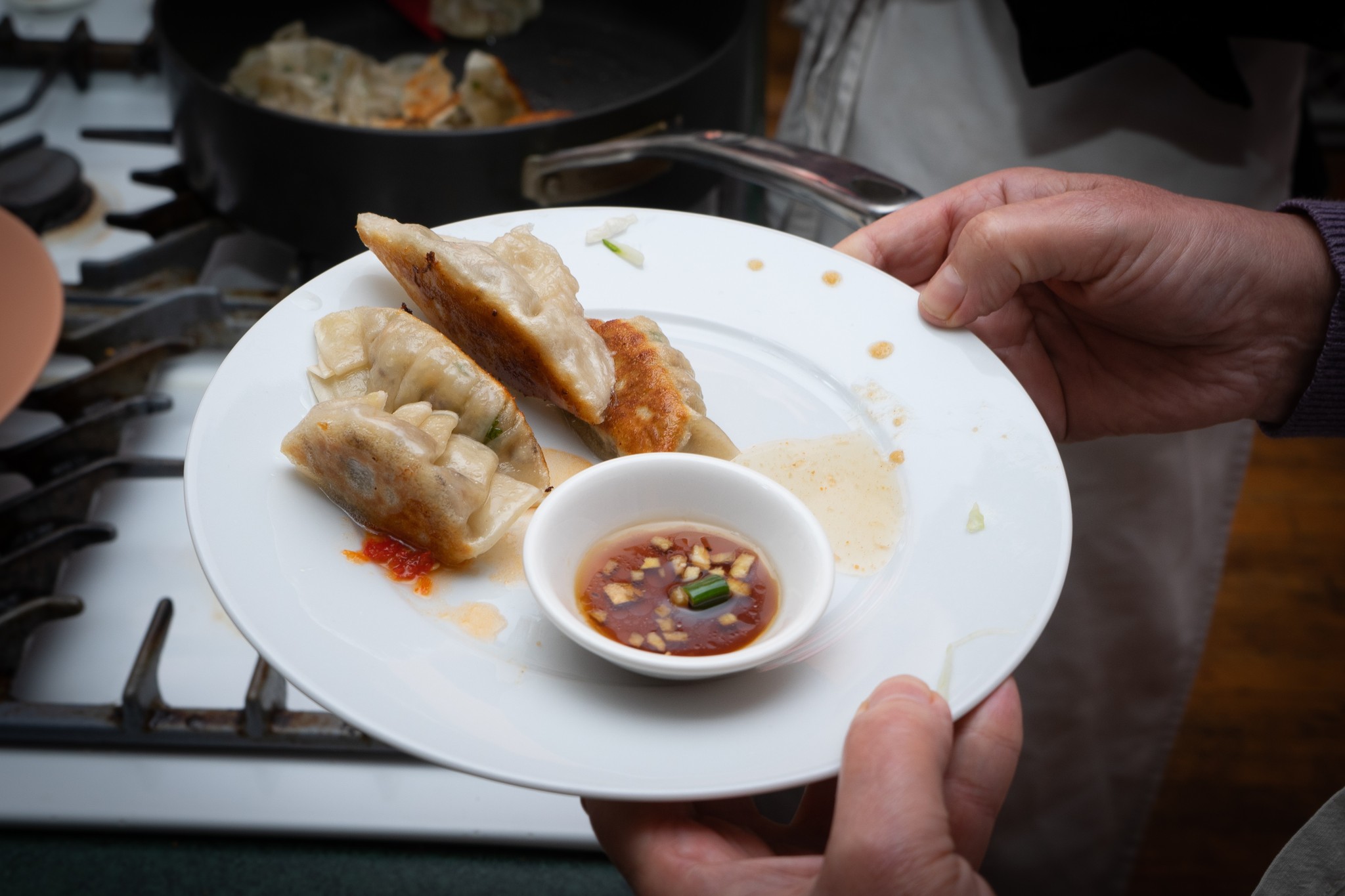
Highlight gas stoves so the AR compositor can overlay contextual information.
[1,0,757,868]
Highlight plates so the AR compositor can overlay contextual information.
[178,200,1077,801]
[1,204,64,423]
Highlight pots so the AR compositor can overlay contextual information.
[149,0,933,227]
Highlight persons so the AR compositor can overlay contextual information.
[579,166,1345,896]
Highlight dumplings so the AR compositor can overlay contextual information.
[575,316,738,462]
[356,212,615,425]
[282,392,540,562]
[310,307,552,505]
[219,1,577,129]
